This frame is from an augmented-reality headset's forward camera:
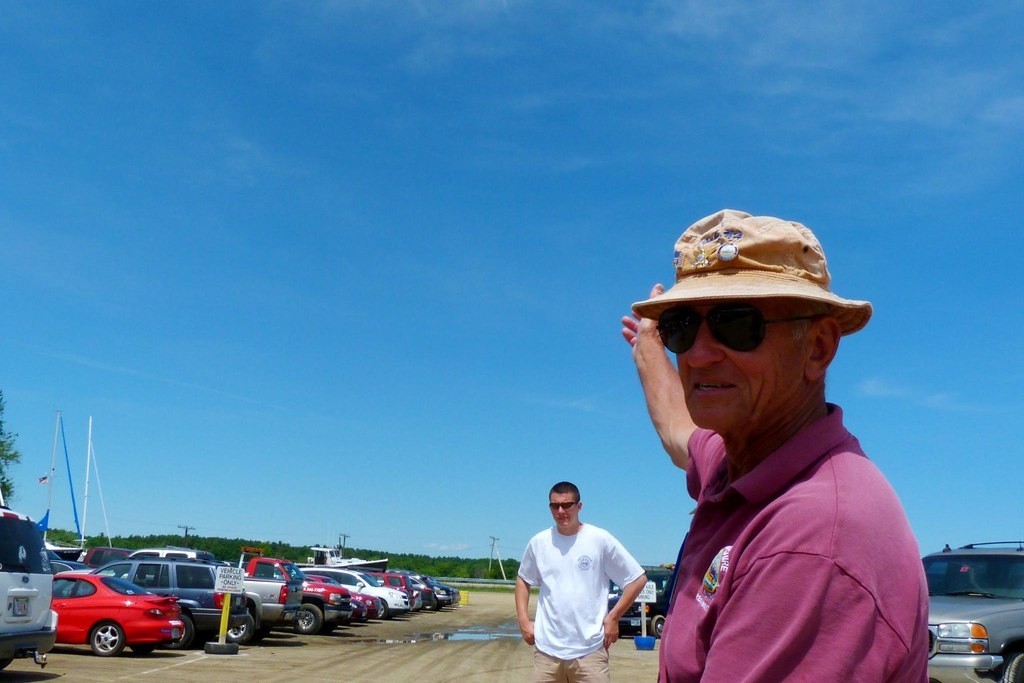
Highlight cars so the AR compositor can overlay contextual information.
[350,599,368,622]
[300,569,410,619]
[91,559,247,650]
[607,570,672,638]
[370,573,414,612]
[211,561,227,566]
[410,591,422,611]
[446,586,461,604]
[407,577,432,610]
[50,573,185,656]
[306,575,381,618]
[48,560,90,574]
[0,505,58,671]
[921,542,1024,683]
[425,578,452,610]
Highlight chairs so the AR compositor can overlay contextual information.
[136,569,148,589]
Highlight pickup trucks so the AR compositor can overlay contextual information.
[223,577,303,646]
[246,557,353,636]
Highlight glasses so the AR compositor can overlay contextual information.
[550,502,578,509]
[656,299,822,354]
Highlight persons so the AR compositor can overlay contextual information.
[621,208,928,683]
[514,481,647,683]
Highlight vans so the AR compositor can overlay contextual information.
[83,547,134,568]
[126,549,216,561]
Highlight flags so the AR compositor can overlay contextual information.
[39,477,48,484]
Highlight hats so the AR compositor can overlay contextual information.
[631,209,872,336]
